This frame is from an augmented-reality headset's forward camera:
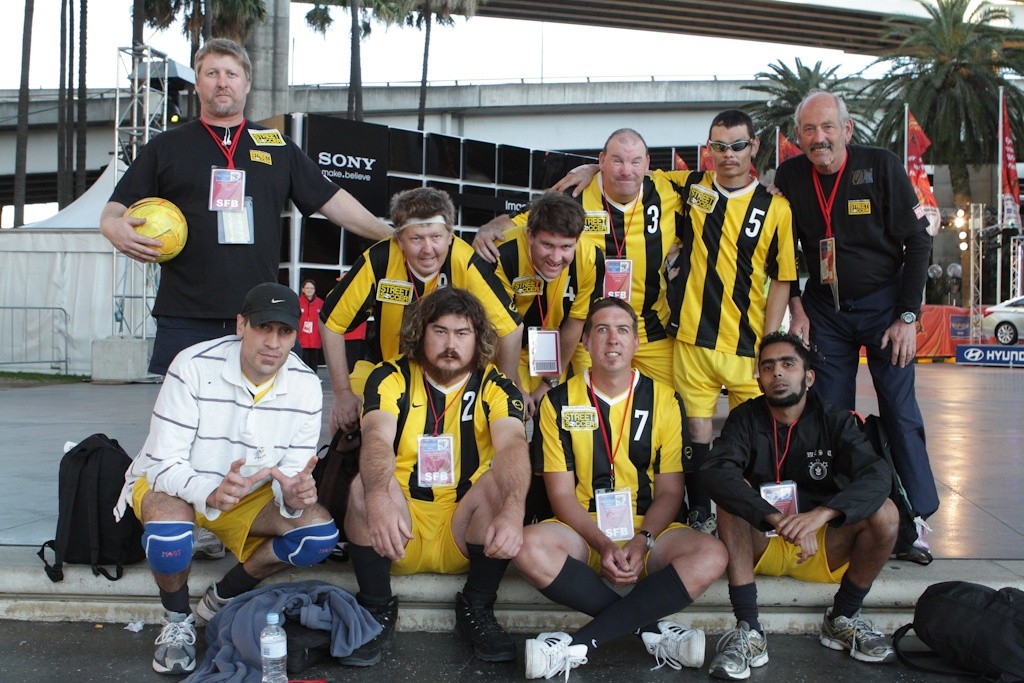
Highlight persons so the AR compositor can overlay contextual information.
[315,186,523,437]
[98,38,941,681]
[703,330,900,680]
[514,296,729,679]
[340,286,532,667]
[112,282,340,676]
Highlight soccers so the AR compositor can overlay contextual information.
[121,198,188,263]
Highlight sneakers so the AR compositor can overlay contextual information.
[709,621,769,681]
[900,518,932,566]
[820,604,896,663]
[639,621,706,671]
[523,631,588,683]
[690,510,718,536]
[454,589,517,661]
[192,525,226,558]
[150,609,196,674]
[340,594,399,667]
[195,583,237,621]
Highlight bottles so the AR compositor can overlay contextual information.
[260,613,288,683]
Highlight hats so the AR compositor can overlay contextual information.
[242,282,302,333]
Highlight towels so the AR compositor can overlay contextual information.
[176,578,384,683]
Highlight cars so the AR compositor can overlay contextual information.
[982,296,1024,345]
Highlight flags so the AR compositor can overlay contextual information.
[1002,95,1022,230]
[778,132,802,164]
[699,146,761,177]
[907,110,939,207]
[673,152,686,169]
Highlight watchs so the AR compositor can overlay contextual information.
[637,530,654,549]
[898,311,917,324]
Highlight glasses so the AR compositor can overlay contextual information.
[709,140,752,151]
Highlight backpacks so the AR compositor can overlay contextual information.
[39,432,148,582]
[851,410,919,552]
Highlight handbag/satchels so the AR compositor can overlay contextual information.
[890,581,1024,683]
